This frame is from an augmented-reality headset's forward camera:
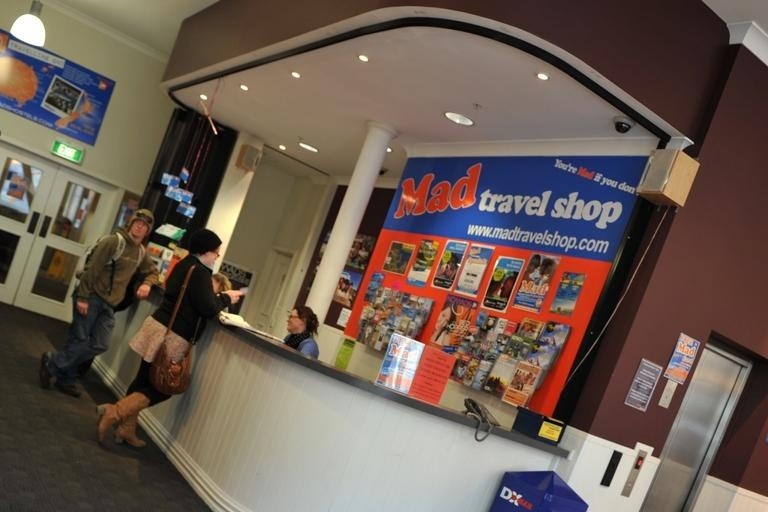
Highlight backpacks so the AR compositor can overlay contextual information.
[76,232,146,278]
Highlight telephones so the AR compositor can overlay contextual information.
[465,398,500,426]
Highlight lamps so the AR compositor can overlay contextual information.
[9,0,46,47]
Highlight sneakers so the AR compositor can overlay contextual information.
[57,383,81,396]
[41,352,50,389]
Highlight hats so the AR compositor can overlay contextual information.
[189,229,222,253]
[128,209,154,236]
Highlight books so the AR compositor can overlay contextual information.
[359,285,433,356]
[144,242,189,289]
[220,310,285,343]
[451,311,571,410]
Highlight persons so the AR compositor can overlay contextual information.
[522,255,540,281]
[533,258,557,286]
[209,272,232,312]
[36,209,159,398]
[283,303,319,358]
[334,277,353,306]
[500,271,519,299]
[486,269,507,298]
[96,230,247,446]
[430,301,459,347]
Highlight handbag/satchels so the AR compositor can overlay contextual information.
[151,343,193,393]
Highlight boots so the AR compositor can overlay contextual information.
[113,412,145,447]
[97,392,149,442]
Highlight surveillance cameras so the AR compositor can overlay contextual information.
[614,116,633,134]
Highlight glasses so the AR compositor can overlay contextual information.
[136,211,152,224]
